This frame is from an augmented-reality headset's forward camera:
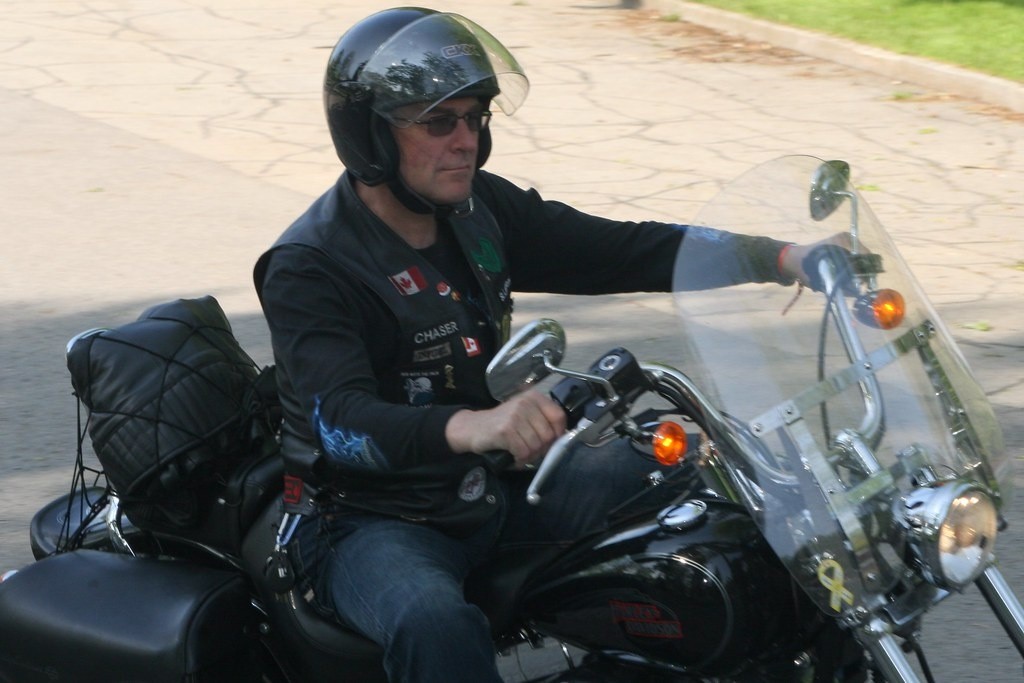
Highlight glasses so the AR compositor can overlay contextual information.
[386,111,491,136]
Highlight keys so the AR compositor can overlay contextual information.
[263,547,296,609]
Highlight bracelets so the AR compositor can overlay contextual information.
[777,242,804,316]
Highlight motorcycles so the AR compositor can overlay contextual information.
[0,153,1024,683]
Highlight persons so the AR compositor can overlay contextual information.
[252,7,872,683]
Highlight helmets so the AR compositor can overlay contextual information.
[324,6,501,186]
